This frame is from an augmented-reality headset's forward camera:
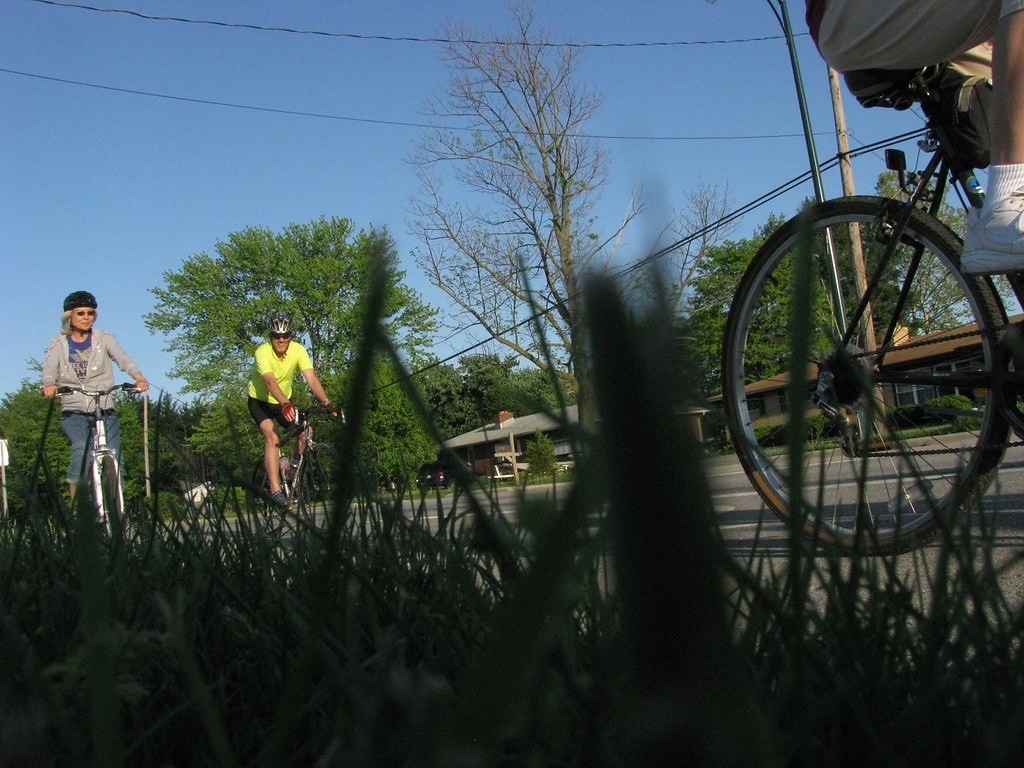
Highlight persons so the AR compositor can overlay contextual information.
[248,310,341,508]
[802,0,1023,275]
[42,290,149,517]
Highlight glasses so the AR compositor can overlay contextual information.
[77,311,95,316]
[270,332,292,339]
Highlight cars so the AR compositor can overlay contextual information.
[414,459,475,491]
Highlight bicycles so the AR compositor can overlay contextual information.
[244,400,351,530]
[41,382,143,549]
[713,64,1024,561]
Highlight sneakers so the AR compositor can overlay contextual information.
[272,491,288,506]
[960,185,1024,276]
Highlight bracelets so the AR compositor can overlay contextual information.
[322,399,328,404]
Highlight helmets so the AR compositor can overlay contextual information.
[268,312,294,333]
[64,291,97,312]
